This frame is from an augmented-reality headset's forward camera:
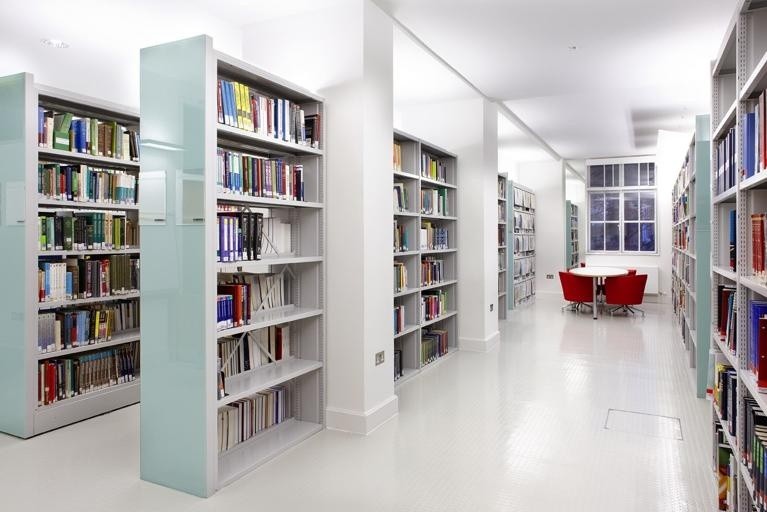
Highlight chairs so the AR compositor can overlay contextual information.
[554,260,652,319]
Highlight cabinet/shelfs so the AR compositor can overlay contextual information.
[135,33,328,494]
[705,1,765,508]
[392,125,459,389]
[668,113,710,399]
[564,200,578,269]
[508,177,538,309]
[494,169,511,323]
[0,70,139,443]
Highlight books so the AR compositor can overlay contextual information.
[217,78,320,453]
[712,88,767,512]
[571,204,578,266]
[38,106,140,408]
[393,142,450,380]
[672,154,690,344]
[498,176,536,303]
